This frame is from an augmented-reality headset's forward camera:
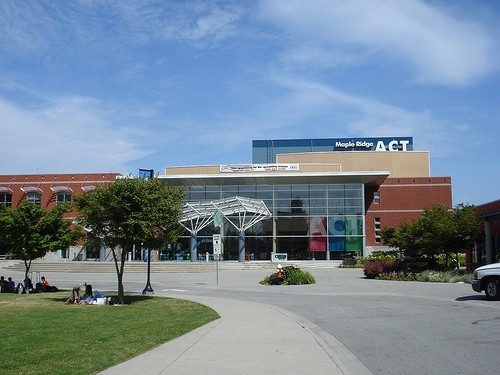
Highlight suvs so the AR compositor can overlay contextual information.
[471,262,500,300]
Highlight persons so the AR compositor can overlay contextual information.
[70,284,93,304]
[0,275,49,293]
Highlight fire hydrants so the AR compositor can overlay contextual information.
[276,264,283,279]
